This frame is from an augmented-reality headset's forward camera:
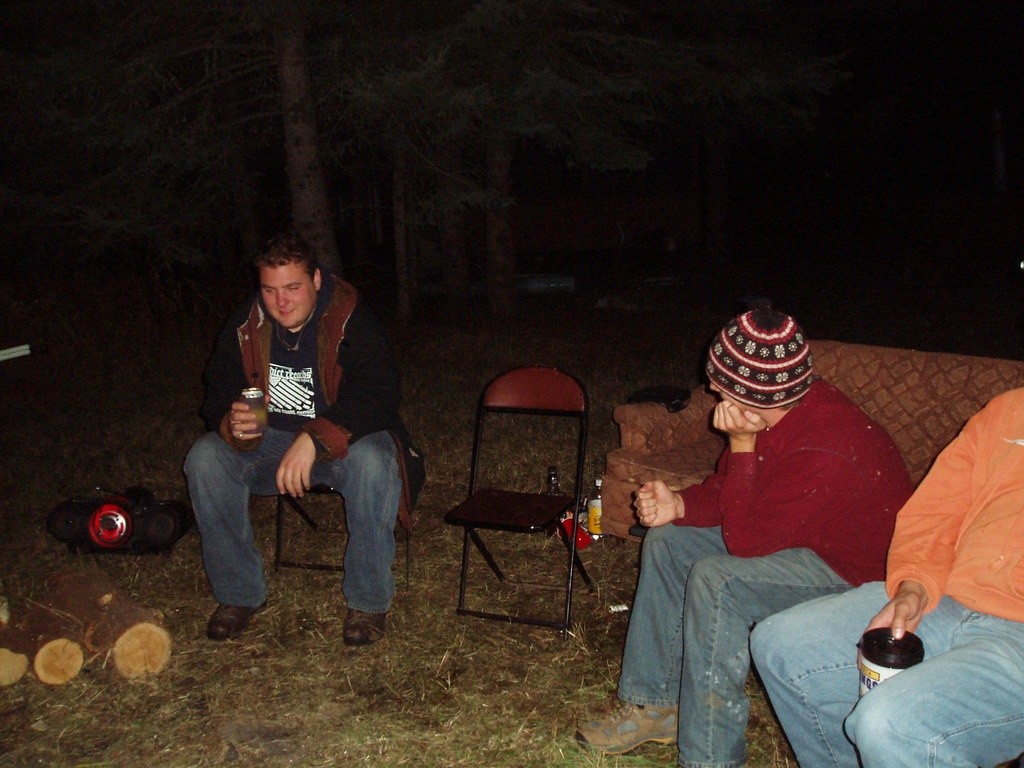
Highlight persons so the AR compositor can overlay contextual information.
[748,386,1024,768]
[182,232,426,646]
[577,300,914,768]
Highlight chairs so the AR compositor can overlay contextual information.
[444,365,586,642]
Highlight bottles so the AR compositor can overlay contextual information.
[542,467,567,497]
[587,478,602,536]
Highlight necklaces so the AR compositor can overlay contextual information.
[274,323,307,352]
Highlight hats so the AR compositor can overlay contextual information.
[705,305,813,409]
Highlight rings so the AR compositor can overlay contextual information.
[239,433,243,439]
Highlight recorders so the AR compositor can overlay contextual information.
[46,485,195,556]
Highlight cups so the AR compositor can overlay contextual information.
[856,626,925,700]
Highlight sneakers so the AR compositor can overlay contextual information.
[575,698,680,755]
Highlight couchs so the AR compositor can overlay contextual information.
[600,340,1024,541]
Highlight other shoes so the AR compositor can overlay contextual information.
[207,595,275,640]
[343,609,386,646]
